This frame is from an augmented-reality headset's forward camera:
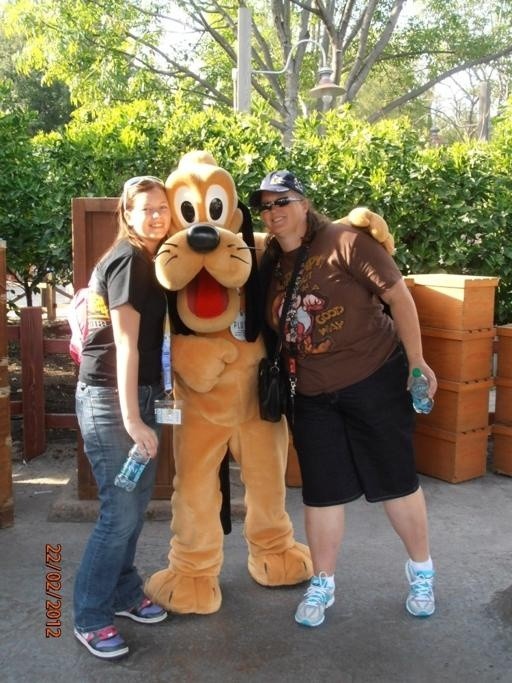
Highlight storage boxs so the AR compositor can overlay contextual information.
[416,383,492,427]
[492,428,512,478]
[411,274,499,336]
[494,324,512,382]
[412,427,489,486]
[493,383,512,427]
[419,336,493,385]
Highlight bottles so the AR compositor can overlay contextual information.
[115,443,152,494]
[406,370,434,413]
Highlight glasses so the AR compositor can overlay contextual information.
[259,197,302,213]
[123,176,164,211]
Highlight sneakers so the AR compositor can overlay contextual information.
[295,571,336,627]
[114,595,167,624]
[74,624,129,658]
[404,560,435,617]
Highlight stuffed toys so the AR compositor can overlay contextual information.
[141,150,395,614]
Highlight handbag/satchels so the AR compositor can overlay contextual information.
[67,288,88,366]
[259,353,288,422]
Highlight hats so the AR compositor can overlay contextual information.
[250,170,304,207]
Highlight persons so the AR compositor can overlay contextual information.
[67,175,172,658]
[248,170,438,627]
